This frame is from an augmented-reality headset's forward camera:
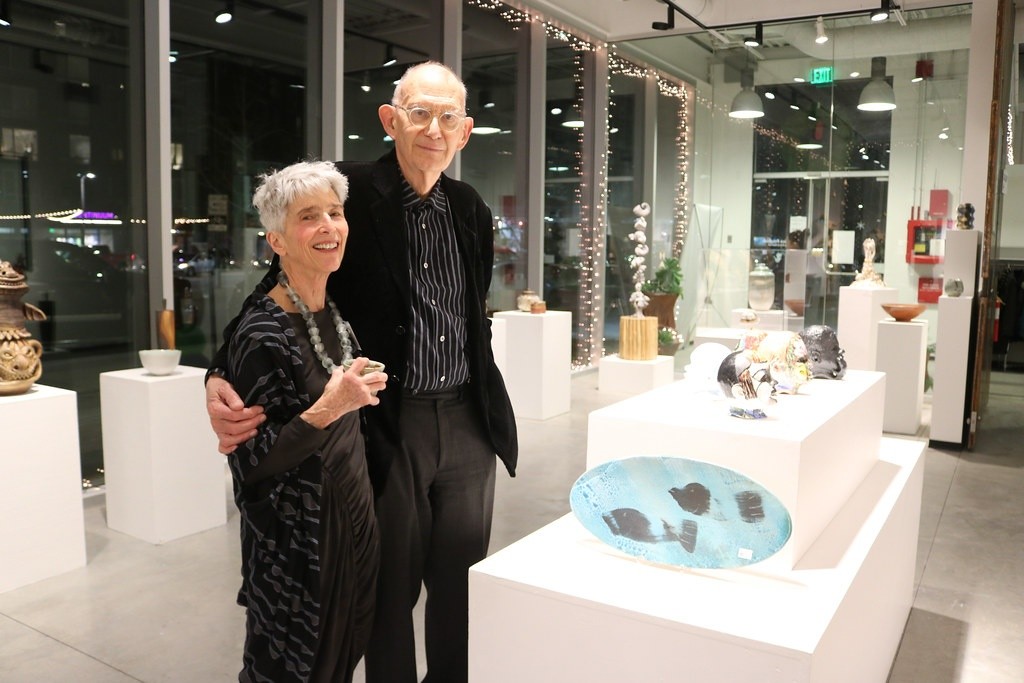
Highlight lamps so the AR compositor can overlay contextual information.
[815,16,829,44]
[869,0,890,21]
[727,46,765,118]
[651,7,674,30]
[744,25,763,47]
[856,57,897,112]
[382,41,396,67]
[216,0,234,24]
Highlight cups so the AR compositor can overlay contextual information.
[343,358,386,396]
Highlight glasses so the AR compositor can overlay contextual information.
[392,103,466,133]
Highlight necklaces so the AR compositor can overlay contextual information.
[278,269,352,375]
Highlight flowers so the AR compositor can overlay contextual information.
[659,326,685,347]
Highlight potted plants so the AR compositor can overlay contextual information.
[629,257,685,329]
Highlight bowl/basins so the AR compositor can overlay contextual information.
[881,303,927,323]
[138,349,182,376]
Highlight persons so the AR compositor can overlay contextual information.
[222,162,390,683]
[767,229,810,310]
[204,60,519,683]
[798,324,847,379]
[758,331,814,395]
[715,348,784,418]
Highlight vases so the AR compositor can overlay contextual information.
[658,343,681,357]
